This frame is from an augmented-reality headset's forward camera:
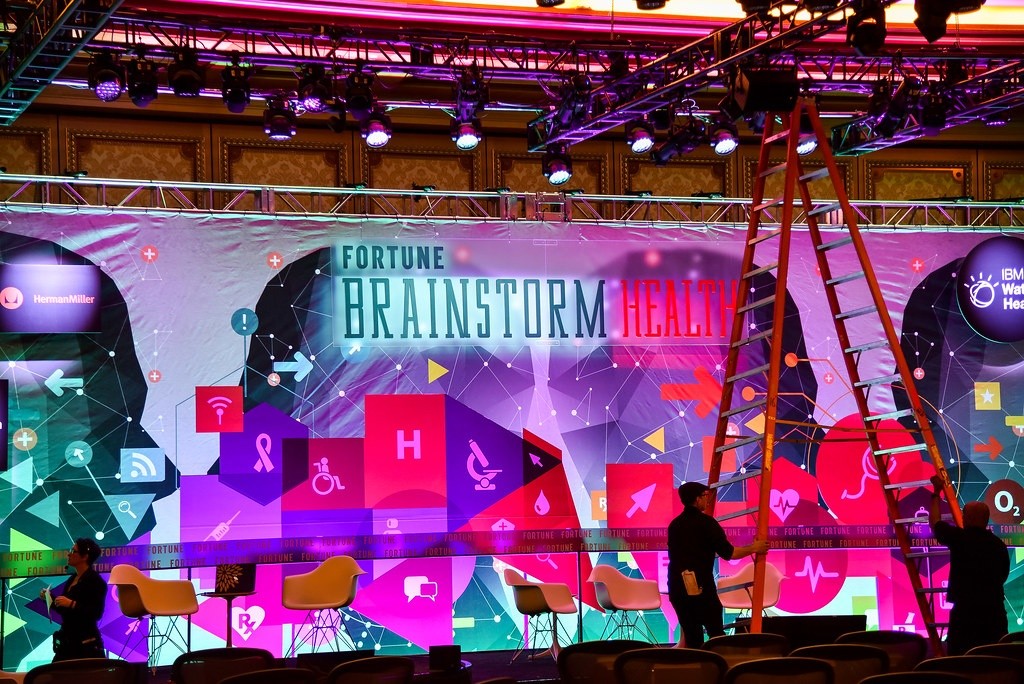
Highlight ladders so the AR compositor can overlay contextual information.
[678,97,964,656]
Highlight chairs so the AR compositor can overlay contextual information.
[24,630,1024,684]
[504,569,578,664]
[107,564,199,664]
[587,565,661,647]
[281,556,367,659]
[717,562,787,618]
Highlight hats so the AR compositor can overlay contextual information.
[678,482,709,499]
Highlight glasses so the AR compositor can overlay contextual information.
[69,548,80,554]
[698,491,710,500]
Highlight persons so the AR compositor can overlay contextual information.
[39,538,107,662]
[928,475,1010,655]
[668,482,769,649]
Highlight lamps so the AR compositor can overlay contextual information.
[83,0,1008,183]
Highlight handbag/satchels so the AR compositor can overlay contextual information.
[52,631,67,655]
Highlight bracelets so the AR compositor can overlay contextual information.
[70,600,74,609]
[930,494,940,498]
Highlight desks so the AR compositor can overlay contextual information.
[200,591,258,647]
